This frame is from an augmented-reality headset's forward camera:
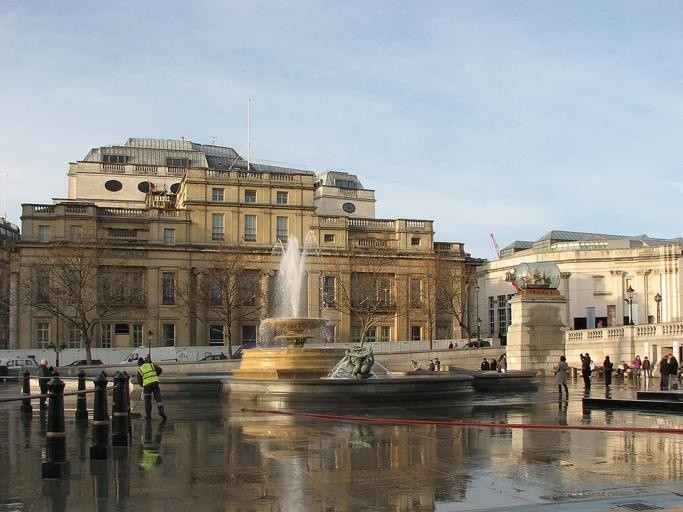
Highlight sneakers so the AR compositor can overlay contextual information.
[40,402,47,406]
[144,414,151,420]
[159,412,167,419]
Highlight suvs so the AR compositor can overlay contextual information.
[0,358,40,376]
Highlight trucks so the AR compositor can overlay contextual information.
[120,346,198,364]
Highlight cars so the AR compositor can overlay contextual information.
[463,341,490,349]
[200,354,228,360]
[64,359,104,369]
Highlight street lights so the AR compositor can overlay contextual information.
[626,285,635,325]
[475,285,482,338]
[654,292,661,323]
[146,328,153,363]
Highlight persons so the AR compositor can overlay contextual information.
[138,421,163,471]
[596,320,604,328]
[37,359,52,411]
[428,360,435,371]
[434,358,440,372]
[553,356,570,397]
[604,356,613,391]
[557,398,569,426]
[449,341,453,349]
[220,352,227,359]
[616,353,683,388]
[580,353,591,398]
[481,354,507,374]
[137,357,167,421]
